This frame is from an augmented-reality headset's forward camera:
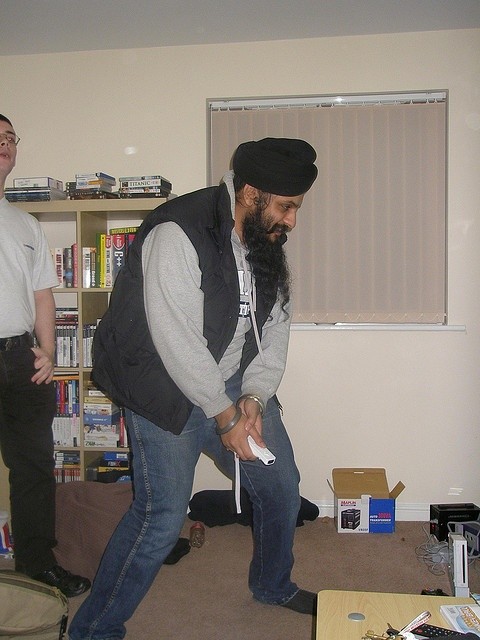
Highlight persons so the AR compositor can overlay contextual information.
[1,115,91,599]
[67,139,318,638]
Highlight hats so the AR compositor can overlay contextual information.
[233,138,318,197]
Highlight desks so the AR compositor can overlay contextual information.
[316,590,480,640]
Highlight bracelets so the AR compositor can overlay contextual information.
[216,406,242,435]
[237,393,265,417]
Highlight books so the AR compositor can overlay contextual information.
[52,307,80,367]
[4,171,172,201]
[50,227,138,288]
[50,370,80,446]
[83,381,128,448]
[53,451,80,483]
[84,451,135,484]
[83,317,103,368]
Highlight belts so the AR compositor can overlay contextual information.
[0,334,34,352]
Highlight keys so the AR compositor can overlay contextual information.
[365,623,406,639]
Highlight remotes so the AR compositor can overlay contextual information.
[240,424,276,466]
[401,621,480,640]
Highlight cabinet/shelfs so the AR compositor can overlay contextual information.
[8,199,167,481]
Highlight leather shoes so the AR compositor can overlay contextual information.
[31,564,91,597]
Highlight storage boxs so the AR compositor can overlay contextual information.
[326,467,406,534]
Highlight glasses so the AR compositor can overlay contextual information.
[0,132,20,146]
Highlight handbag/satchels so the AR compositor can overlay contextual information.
[0,568,70,640]
